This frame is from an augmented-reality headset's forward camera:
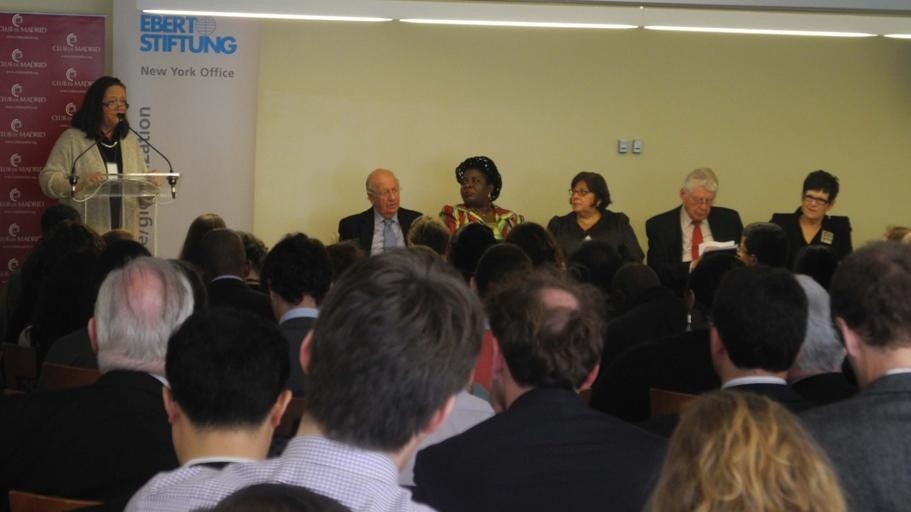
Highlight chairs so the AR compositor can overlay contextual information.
[0,337,108,511]
[649,387,698,416]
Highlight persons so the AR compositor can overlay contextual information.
[336,168,425,254]
[439,155,526,240]
[770,168,853,259]
[1,215,908,512]
[546,170,647,282]
[644,166,743,265]
[37,75,164,244]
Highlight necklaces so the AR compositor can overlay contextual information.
[98,140,120,148]
[578,215,594,226]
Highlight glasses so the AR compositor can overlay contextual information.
[569,188,590,196]
[803,195,828,205]
[102,102,128,111]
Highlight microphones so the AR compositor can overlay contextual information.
[116,112,174,172]
[71,120,127,176]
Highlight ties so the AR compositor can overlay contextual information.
[383,219,396,247]
[691,219,704,261]
